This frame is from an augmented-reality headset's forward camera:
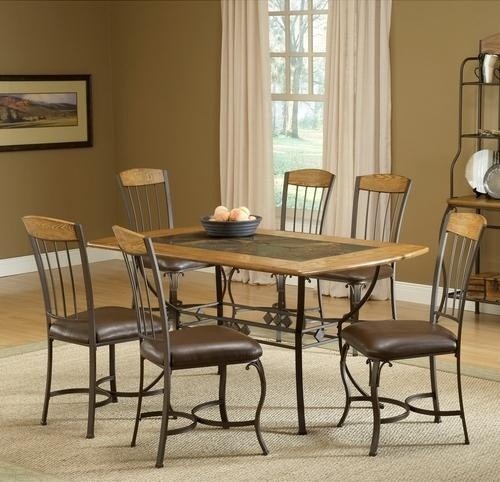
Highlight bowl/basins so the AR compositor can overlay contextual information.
[200,215,262,237]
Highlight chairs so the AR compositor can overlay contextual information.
[336,212,487,456]
[21,215,177,439]
[238,169,334,341]
[116,168,224,329]
[302,174,411,356]
[111,225,269,467]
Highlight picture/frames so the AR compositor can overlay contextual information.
[0,74,94,153]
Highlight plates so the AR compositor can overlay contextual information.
[464,149,499,194]
[483,163,500,199]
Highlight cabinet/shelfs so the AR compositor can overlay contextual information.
[436,32,500,328]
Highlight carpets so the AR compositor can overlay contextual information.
[0,318,500,482]
